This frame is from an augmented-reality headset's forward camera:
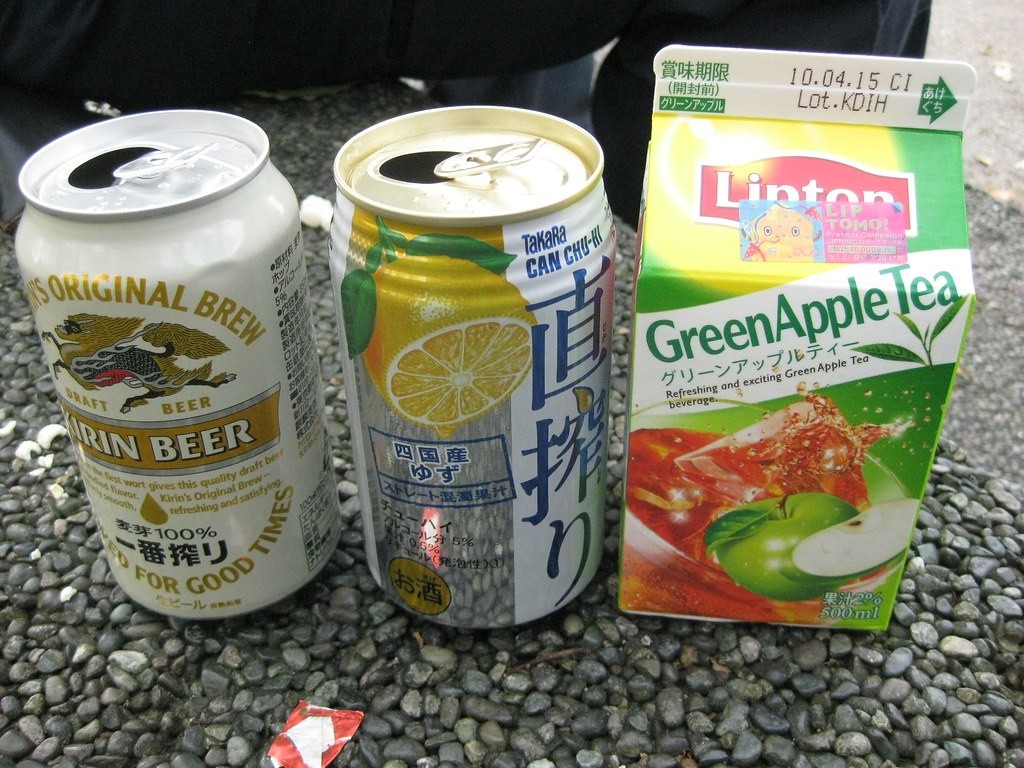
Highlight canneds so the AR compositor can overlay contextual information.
[15,107,620,630]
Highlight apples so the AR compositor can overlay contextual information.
[704,492,918,601]
